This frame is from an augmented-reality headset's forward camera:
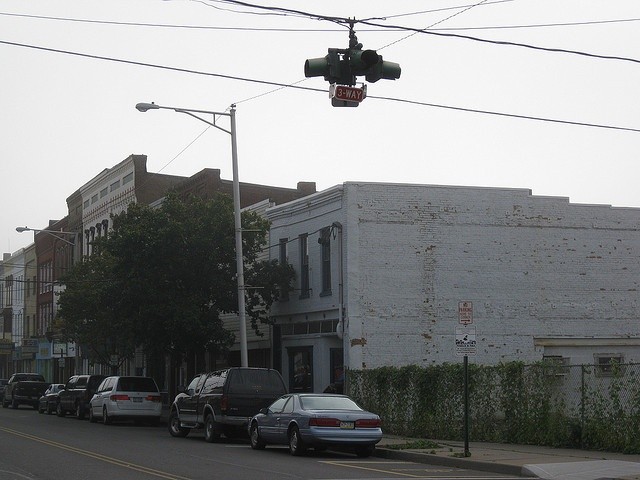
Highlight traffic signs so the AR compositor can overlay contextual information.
[458,300,474,324]
[454,324,477,357]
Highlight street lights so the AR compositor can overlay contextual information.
[16,226,78,248]
[135,100,250,367]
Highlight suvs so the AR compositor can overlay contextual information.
[168,368,286,444]
[54,374,104,420]
[88,375,162,426]
[1,371,46,409]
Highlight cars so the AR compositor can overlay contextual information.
[247,391,384,457]
[37,384,67,416]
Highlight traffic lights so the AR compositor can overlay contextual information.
[304,45,402,86]
